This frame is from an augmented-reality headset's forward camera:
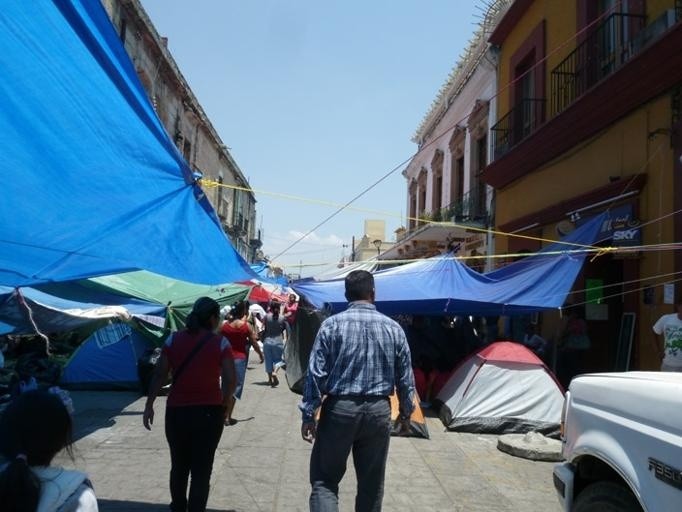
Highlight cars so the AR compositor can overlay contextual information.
[553,367,682,512]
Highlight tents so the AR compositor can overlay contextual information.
[433,341,565,433]
[56,323,153,389]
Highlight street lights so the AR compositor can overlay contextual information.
[373,239,381,255]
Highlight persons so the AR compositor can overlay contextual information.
[650,296,682,372]
[296,269,415,511]
[0,333,39,412]
[556,302,594,389]
[402,313,550,372]
[1,389,99,512]
[142,292,298,511]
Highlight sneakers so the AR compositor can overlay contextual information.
[268,372,279,387]
